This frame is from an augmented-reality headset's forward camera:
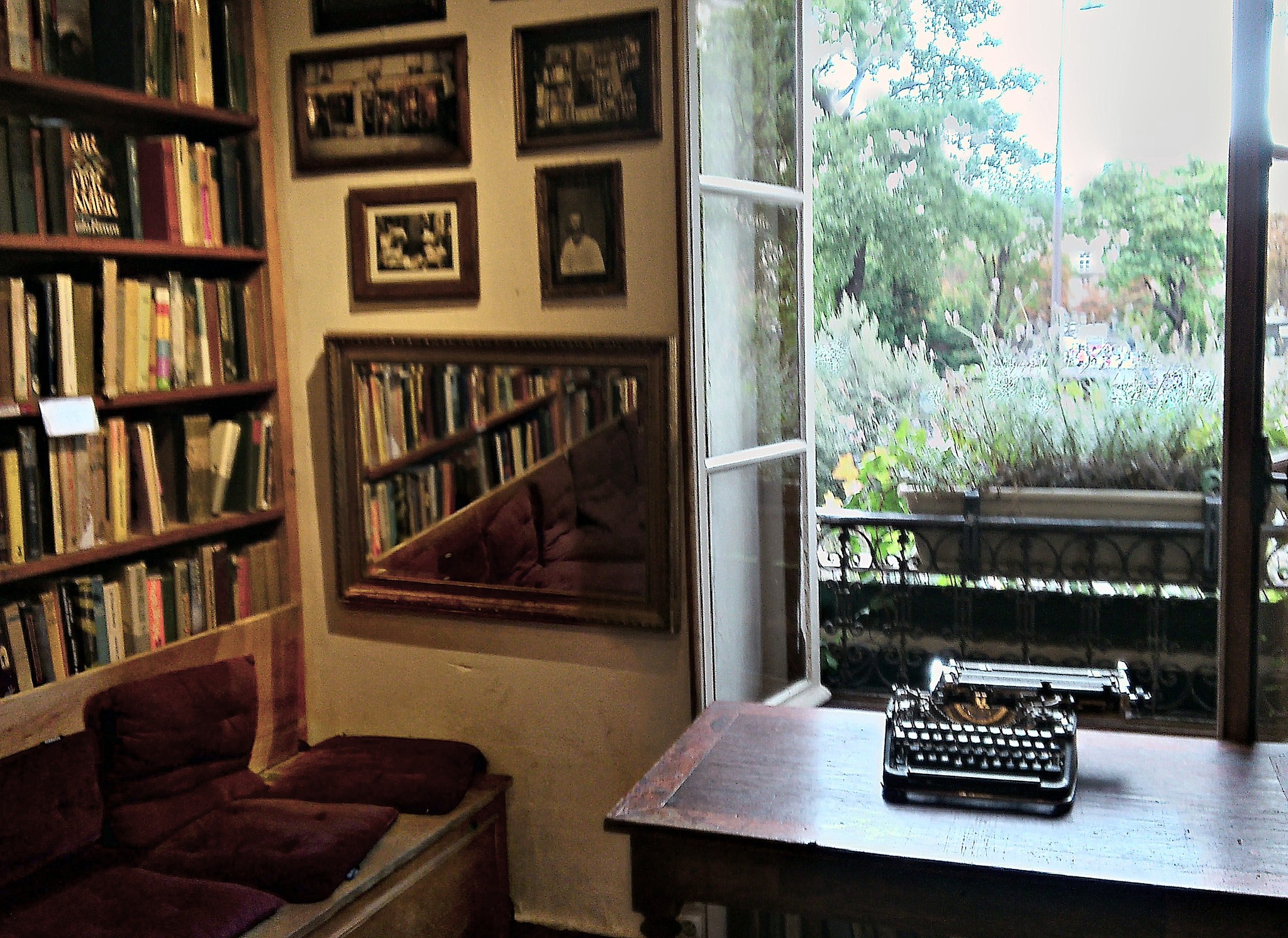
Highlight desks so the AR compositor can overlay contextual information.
[605,701,1288,938]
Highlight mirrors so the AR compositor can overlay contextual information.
[324,328,677,637]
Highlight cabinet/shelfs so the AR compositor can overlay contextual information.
[362,366,643,572]
[0,0,308,775]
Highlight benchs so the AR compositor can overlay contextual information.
[232,754,515,938]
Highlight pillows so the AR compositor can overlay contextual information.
[1,737,105,884]
[394,422,645,583]
[82,653,274,846]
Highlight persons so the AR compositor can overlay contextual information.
[312,51,638,274]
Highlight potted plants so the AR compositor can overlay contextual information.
[819,370,1288,587]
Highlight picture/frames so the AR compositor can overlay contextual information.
[348,181,480,302]
[512,7,662,154]
[289,34,471,174]
[314,0,449,35]
[535,158,628,301]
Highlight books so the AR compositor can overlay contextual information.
[361,363,644,563]
[0,0,289,700]
